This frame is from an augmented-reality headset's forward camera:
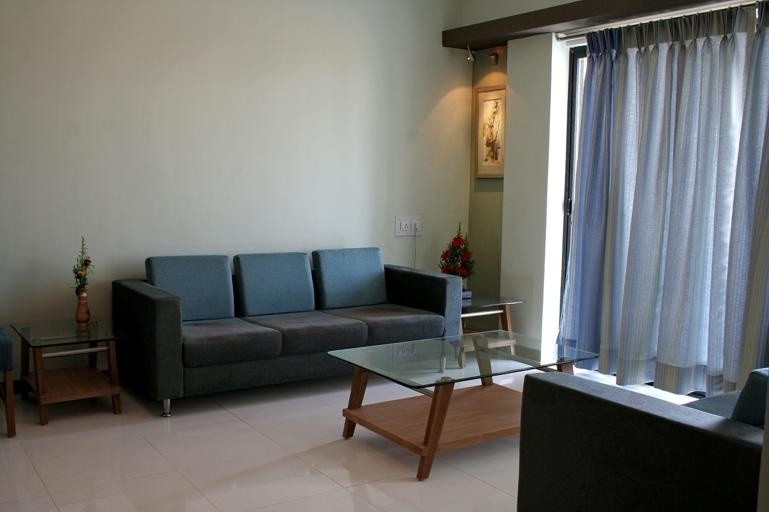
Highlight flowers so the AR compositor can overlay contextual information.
[435,221,477,281]
[72,234,97,295]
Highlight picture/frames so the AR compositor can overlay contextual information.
[470,83,508,182]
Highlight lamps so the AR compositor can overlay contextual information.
[462,42,499,68]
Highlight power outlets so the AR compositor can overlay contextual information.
[394,216,423,236]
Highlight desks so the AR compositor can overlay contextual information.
[10,314,125,427]
[459,292,527,368]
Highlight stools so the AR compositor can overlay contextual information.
[1,326,21,441]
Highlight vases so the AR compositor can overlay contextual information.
[461,277,469,293]
[75,286,92,326]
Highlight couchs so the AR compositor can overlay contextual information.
[105,242,473,422]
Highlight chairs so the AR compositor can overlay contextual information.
[509,352,769,511]
